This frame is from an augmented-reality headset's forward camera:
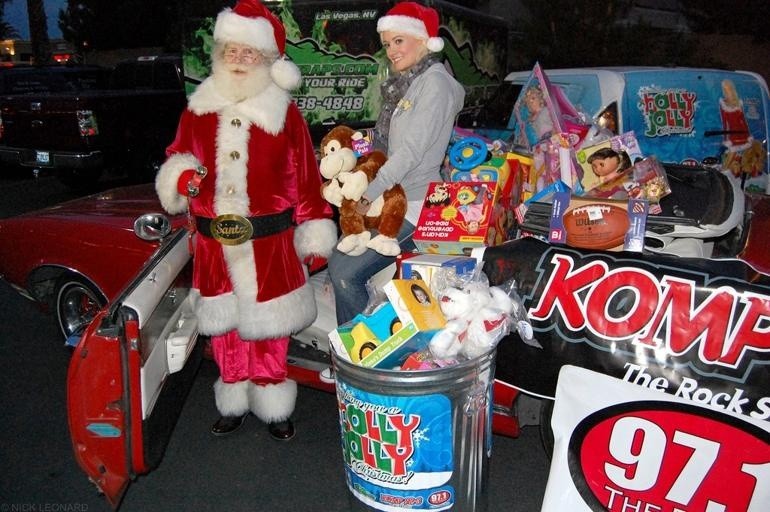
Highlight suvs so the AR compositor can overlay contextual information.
[1,63,115,97]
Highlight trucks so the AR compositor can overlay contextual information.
[469,66,770,190]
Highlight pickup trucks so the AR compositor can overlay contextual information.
[1,85,186,193]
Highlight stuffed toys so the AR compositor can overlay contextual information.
[319,124,408,257]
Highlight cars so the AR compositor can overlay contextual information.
[1,163,770,512]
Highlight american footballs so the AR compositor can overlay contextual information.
[563,204,629,249]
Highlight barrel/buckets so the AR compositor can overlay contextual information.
[332,347,497,512]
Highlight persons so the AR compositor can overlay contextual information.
[523,86,553,139]
[587,147,632,188]
[317,1,465,384]
[412,286,429,305]
[719,80,756,153]
[154,1,339,441]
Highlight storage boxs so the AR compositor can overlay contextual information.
[322,179,517,372]
[546,190,649,255]
